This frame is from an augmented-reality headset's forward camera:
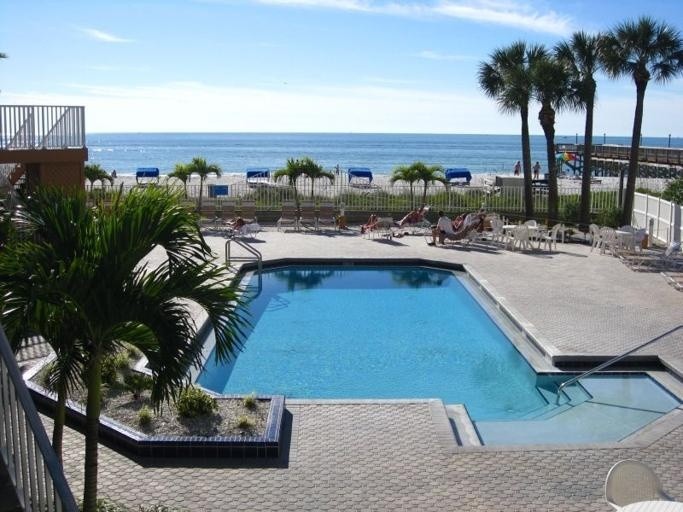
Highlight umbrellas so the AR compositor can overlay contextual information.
[555,150,579,172]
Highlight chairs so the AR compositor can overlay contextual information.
[195,199,683,272]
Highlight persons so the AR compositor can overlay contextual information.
[514,161,521,177]
[335,164,339,175]
[233,217,257,230]
[361,217,384,233]
[533,161,540,179]
[395,204,486,246]
